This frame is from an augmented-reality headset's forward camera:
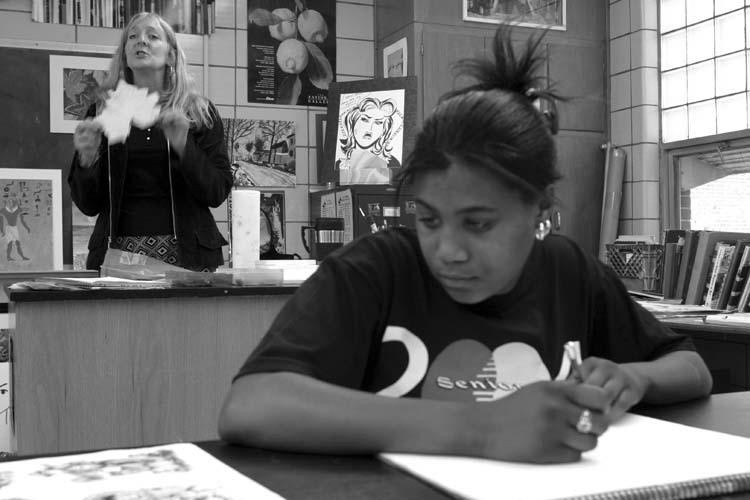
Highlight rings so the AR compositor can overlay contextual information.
[573,405,593,437]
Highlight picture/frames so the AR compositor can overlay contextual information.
[0,305,19,457]
[381,37,411,79]
[45,52,117,136]
[315,113,330,186]
[0,162,68,274]
[321,73,419,199]
[227,188,286,261]
[460,0,571,34]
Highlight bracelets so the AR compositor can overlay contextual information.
[79,149,99,168]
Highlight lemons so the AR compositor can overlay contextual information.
[266,8,329,77]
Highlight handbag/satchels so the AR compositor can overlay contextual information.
[110,235,181,266]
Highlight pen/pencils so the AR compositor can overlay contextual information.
[358,200,387,234]
[564,345,584,383]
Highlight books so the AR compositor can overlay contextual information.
[376,411,750,500]
[31,0,214,35]
[663,229,750,313]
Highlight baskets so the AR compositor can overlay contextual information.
[605,244,662,278]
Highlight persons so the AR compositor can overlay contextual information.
[336,97,401,184]
[67,13,233,272]
[2,198,31,262]
[218,16,715,463]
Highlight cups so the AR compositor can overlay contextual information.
[326,182,336,190]
[299,218,346,263]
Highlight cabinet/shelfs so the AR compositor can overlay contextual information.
[308,187,416,249]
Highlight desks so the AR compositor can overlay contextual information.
[0,275,315,460]
[632,298,749,393]
[0,388,750,498]
